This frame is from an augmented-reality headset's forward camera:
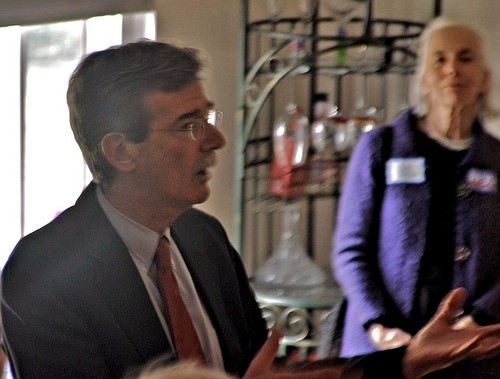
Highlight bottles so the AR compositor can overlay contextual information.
[338,106,376,194]
[271,104,309,197]
[310,104,346,195]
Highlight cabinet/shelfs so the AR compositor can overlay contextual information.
[232,0,442,358]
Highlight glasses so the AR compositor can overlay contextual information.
[122,111,223,141]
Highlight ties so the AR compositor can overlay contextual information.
[157,237,207,367]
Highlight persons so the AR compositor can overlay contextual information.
[1,37,500,379]
[131,354,236,379]
[332,16,499,357]
